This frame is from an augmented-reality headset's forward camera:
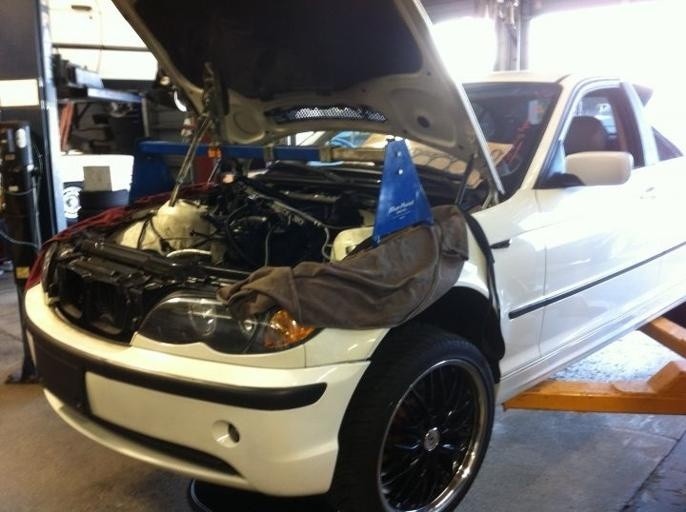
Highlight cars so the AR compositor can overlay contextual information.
[17,2,686,509]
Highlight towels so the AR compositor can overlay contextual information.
[217,201,469,332]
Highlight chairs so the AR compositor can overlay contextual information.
[563,117,610,158]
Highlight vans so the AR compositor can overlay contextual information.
[47,83,188,225]
